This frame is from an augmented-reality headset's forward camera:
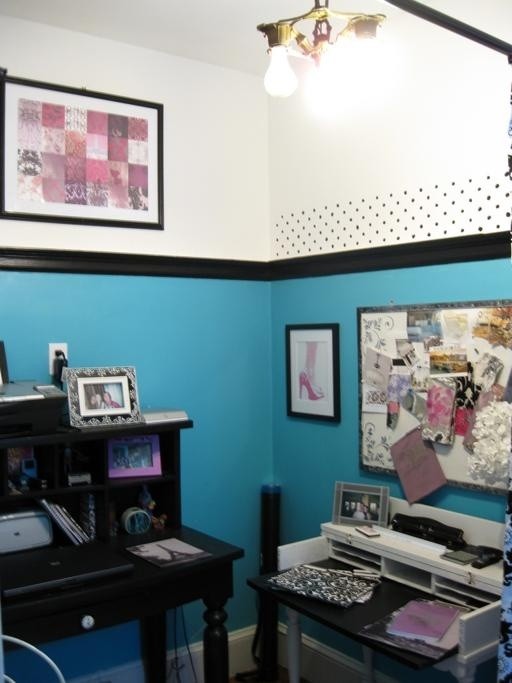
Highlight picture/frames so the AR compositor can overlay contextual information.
[285,323,341,422]
[0,75,165,232]
[64,366,142,429]
[330,480,390,529]
[107,433,163,479]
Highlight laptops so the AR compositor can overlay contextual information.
[0,545,133,604]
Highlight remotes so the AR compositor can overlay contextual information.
[472,554,500,569]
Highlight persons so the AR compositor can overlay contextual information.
[353,503,373,521]
[299,342,325,401]
[88,392,121,409]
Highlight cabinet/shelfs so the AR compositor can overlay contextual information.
[0,407,194,558]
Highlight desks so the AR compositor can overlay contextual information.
[247,497,504,683]
[0,525,247,681]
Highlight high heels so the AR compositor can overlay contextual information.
[299,371,324,400]
